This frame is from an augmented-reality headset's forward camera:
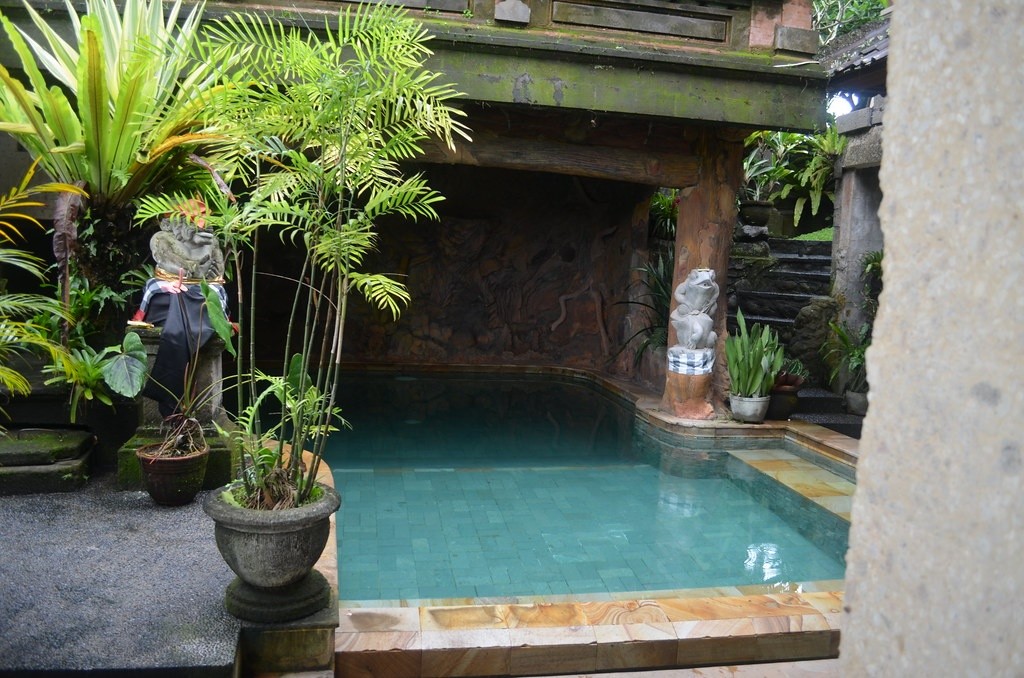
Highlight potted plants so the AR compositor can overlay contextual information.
[603,241,675,395]
[762,358,810,421]
[724,307,788,424]
[818,321,872,417]
[0,1,478,628]
[740,147,795,227]
